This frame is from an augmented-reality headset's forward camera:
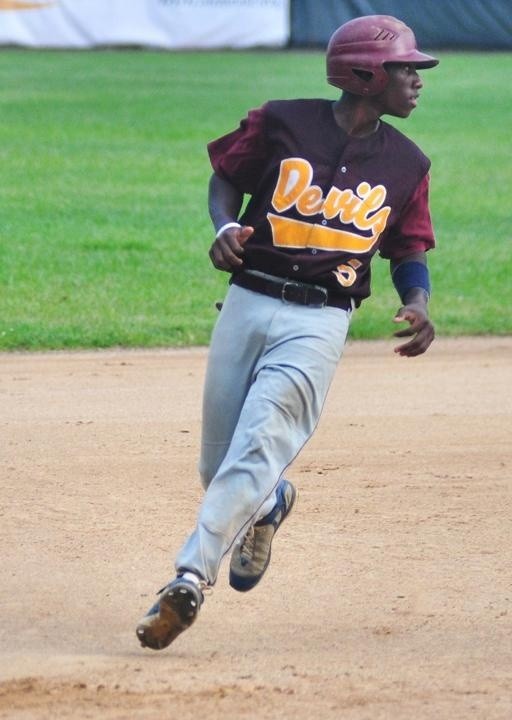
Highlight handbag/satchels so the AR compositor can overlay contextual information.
[215,222,242,238]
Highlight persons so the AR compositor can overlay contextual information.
[136,13,439,651]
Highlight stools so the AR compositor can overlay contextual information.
[228,266,355,312]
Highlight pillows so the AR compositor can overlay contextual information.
[326,15,439,98]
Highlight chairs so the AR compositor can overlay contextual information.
[136,578,204,650]
[229,479,296,592]
